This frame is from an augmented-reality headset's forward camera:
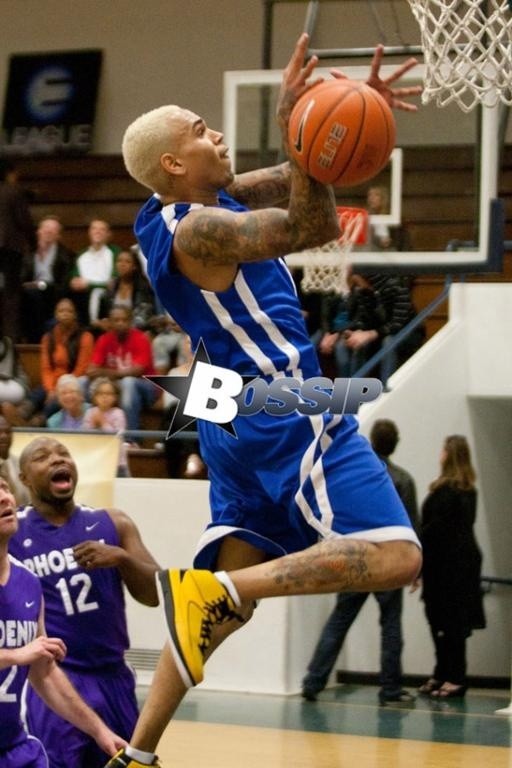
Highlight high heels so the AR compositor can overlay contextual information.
[417,679,468,704]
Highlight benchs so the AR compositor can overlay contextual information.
[0,142,512,481]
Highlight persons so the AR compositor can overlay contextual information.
[300,419,419,708]
[2,414,29,514]
[102,33,424,766]
[414,433,487,697]
[9,436,166,766]
[1,477,129,767]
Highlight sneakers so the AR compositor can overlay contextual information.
[102,747,162,768]
[154,567,241,693]
[493,701,512,717]
[303,686,318,703]
[377,693,415,707]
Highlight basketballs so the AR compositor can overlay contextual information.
[287,78,396,188]
